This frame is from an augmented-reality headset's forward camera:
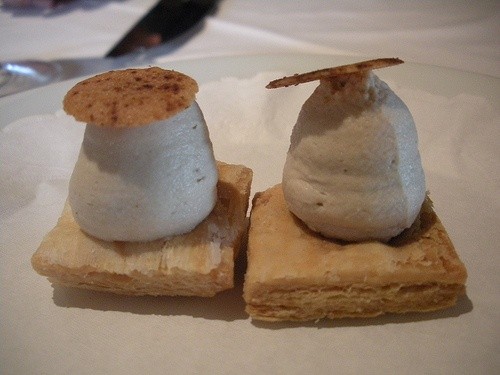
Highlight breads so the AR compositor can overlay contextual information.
[30,67,254,299]
[241,53,467,324]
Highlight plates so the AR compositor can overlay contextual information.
[1,49,499,375]
